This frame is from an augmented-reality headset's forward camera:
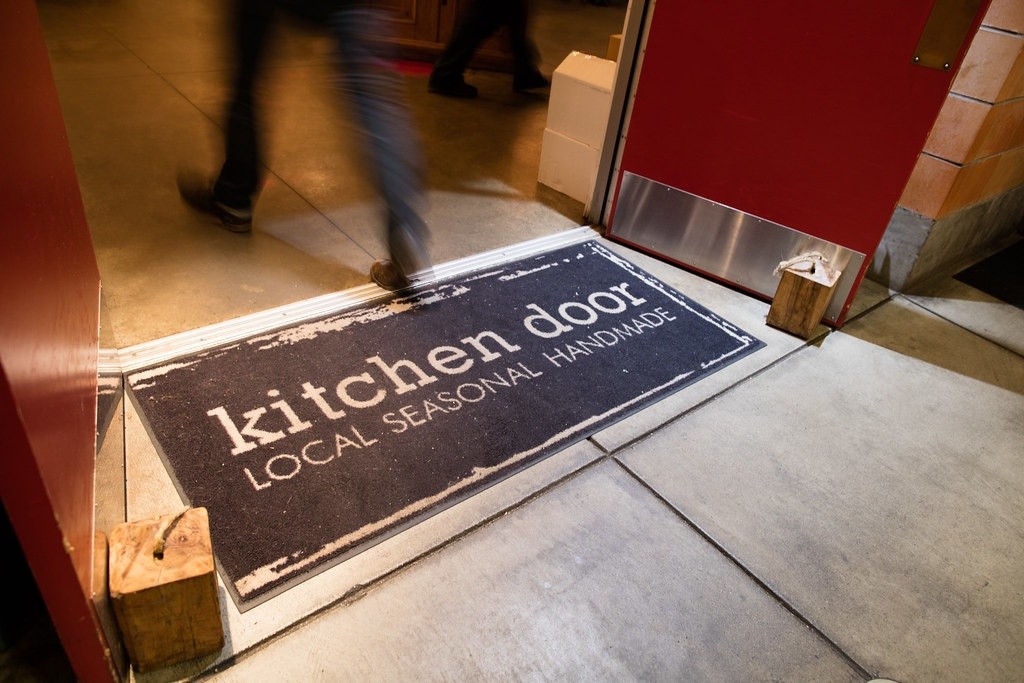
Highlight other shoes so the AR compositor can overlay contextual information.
[512,76,550,96]
[430,79,478,99]
[371,258,418,291]
[176,167,251,232]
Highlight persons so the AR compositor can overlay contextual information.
[177,0,436,290]
[428,0,551,99]
